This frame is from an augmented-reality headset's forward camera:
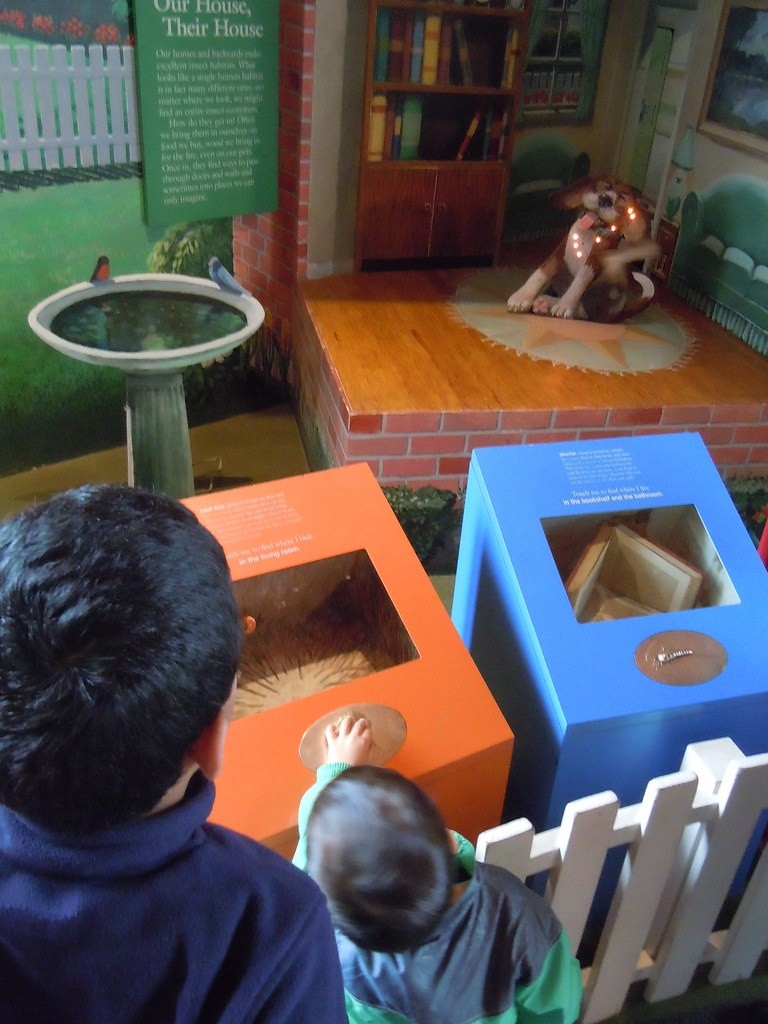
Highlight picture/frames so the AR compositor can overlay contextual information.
[696,0,768,162]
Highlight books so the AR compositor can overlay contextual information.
[376,7,472,86]
[501,24,519,89]
[367,94,422,160]
[455,99,510,160]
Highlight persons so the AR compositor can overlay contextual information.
[292,716,581,1023]
[0,483,348,1024]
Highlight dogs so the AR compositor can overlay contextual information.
[505,174,664,322]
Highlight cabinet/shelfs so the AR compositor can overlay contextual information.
[354,0,532,272]
